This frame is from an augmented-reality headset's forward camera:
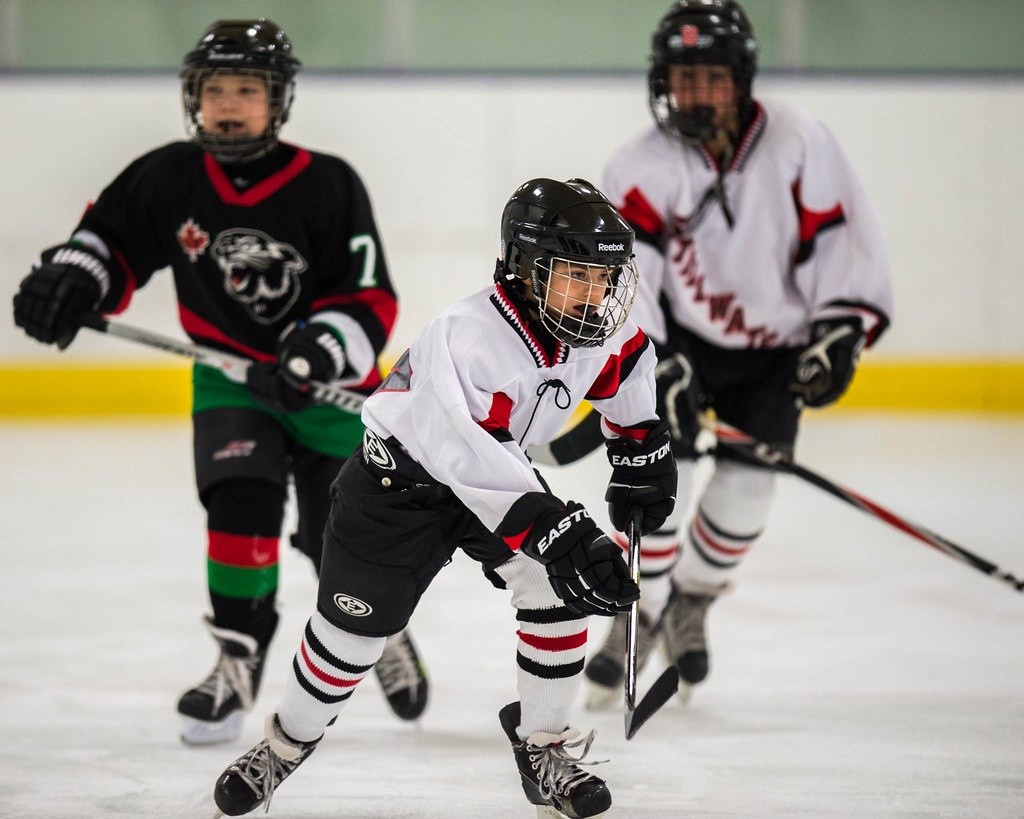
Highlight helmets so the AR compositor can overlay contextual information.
[647,0,757,146]
[178,17,303,164]
[493,178,640,348]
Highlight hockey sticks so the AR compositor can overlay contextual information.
[77,308,366,415]
[713,416,1024,598]
[622,512,683,741]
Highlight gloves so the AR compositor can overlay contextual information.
[521,500,641,617]
[788,315,864,408]
[12,241,116,352]
[247,320,347,416]
[605,420,678,538]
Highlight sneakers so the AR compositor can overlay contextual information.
[498,701,612,819]
[584,577,734,714]
[178,610,280,746]
[374,629,430,722]
[213,712,339,819]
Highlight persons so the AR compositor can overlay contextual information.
[582,0,894,713]
[213,177,679,819]
[12,18,431,750]
